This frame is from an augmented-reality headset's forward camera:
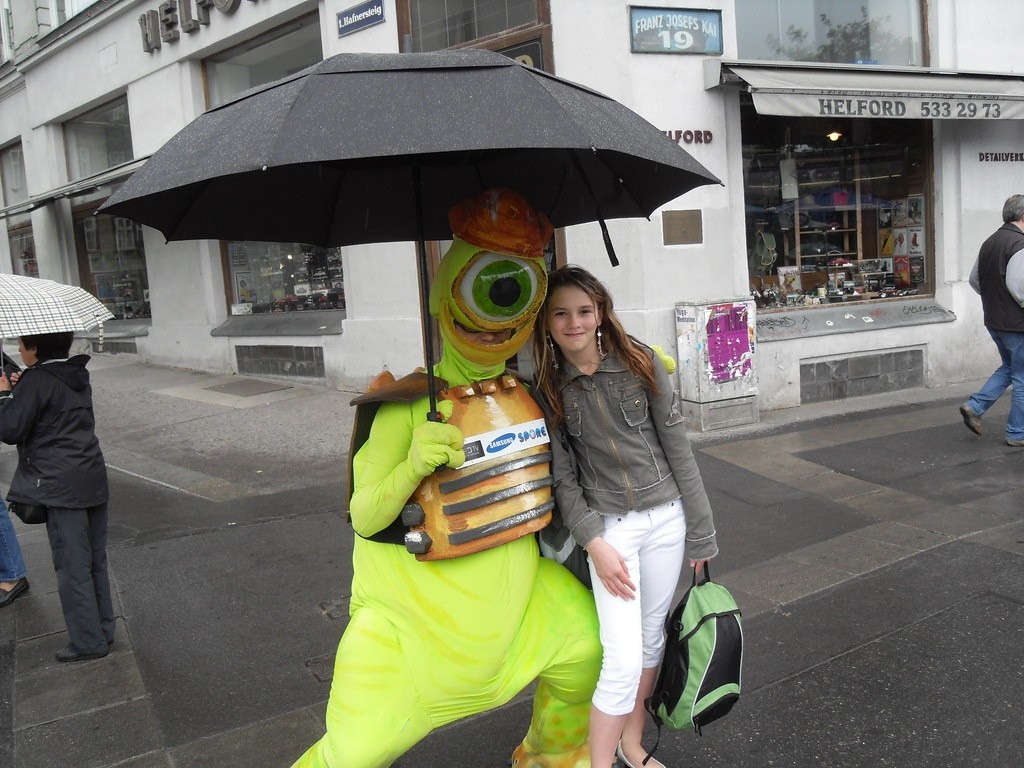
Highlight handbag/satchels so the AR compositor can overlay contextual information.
[7,502,48,524]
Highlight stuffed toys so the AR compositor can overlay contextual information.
[290,188,602,768]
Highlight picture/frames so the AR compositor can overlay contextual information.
[234,271,260,303]
[94,273,116,300]
[89,254,118,273]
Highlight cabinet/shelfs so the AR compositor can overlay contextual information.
[743,150,863,292]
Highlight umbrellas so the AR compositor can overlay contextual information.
[0,274,116,377]
[91,34,725,475]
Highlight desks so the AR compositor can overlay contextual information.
[828,289,896,300]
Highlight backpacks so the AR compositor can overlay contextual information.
[651,560,744,738]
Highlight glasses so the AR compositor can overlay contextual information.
[453,318,482,333]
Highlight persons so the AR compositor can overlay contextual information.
[529,263,718,768]
[960,195,1024,447]
[0,332,115,661]
[240,280,253,301]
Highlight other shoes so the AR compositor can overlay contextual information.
[55,642,110,662]
[959,403,984,436]
[0,577,30,608]
[617,739,666,768]
[107,639,114,645]
[1006,436,1024,446]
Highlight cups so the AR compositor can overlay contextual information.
[817,288,827,298]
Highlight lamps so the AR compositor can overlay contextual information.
[827,131,842,141]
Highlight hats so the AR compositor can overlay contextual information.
[448,185,554,260]
